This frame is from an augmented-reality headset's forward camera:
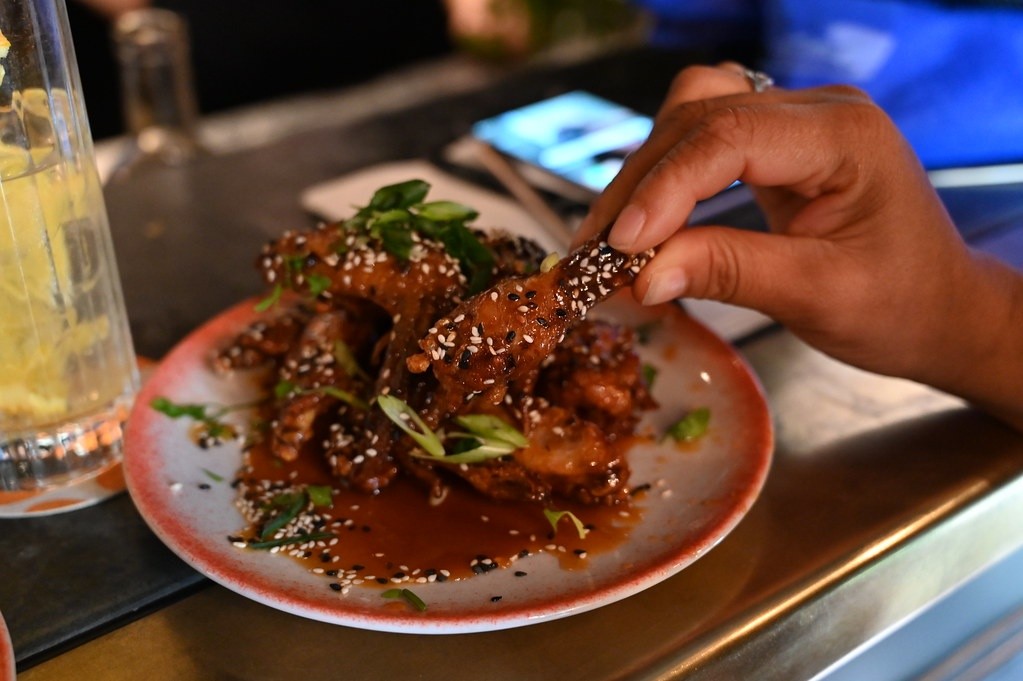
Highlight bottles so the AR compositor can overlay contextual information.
[2,0,139,489]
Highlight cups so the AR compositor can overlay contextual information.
[118,12,198,165]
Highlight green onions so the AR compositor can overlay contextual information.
[347,180,494,294]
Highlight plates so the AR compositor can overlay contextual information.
[122,284,775,635]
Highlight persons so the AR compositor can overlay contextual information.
[566,62,1022,438]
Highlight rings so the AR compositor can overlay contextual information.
[746,69,775,93]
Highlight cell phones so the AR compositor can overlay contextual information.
[467,88,756,229]
